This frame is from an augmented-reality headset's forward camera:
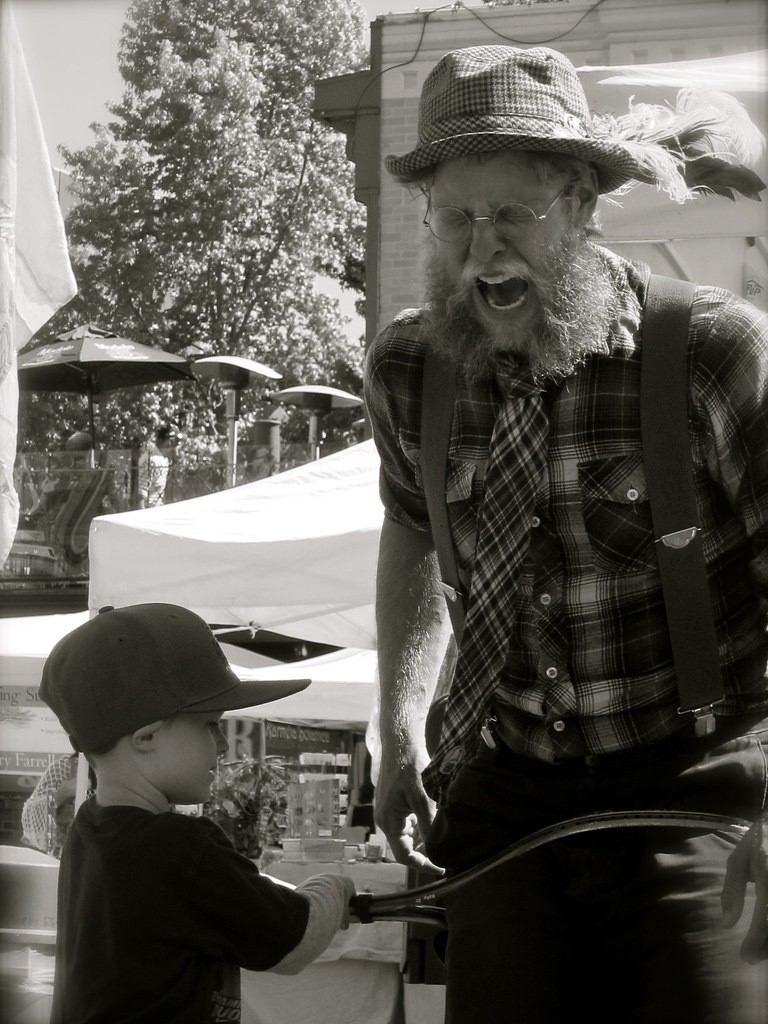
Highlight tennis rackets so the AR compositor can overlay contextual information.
[348,811,763,927]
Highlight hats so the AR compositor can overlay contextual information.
[38,603,311,752]
[384,45,639,194]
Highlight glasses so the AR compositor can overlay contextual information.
[423,186,568,242]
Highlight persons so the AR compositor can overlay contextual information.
[40,603,356,1024]
[362,46,768,1024]
[67,422,93,460]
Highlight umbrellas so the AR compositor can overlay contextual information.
[17,323,186,450]
